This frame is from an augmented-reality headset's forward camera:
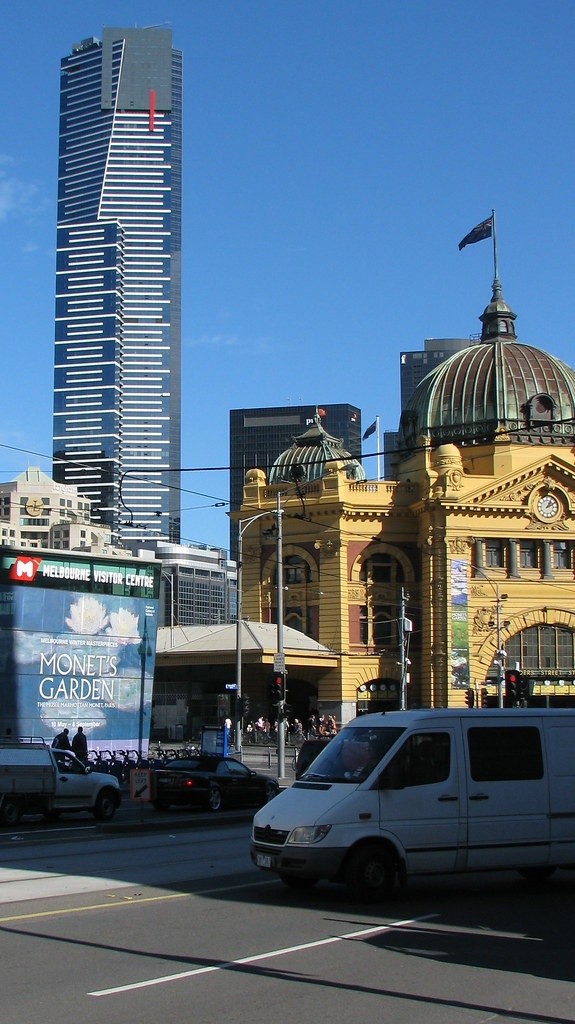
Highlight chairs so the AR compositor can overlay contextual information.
[407,740,441,785]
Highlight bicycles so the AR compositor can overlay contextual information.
[59,741,241,785]
[228,729,307,745]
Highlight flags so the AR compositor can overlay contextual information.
[457,215,493,249]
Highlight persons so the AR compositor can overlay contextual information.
[347,738,385,781]
[224,714,336,743]
[71,727,88,769]
[52,729,72,762]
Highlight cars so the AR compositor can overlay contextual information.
[150,756,280,811]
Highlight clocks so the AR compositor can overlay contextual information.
[537,495,559,518]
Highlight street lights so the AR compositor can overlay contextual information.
[278,464,357,776]
[235,509,284,751]
[471,566,504,709]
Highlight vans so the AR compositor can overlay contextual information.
[247,710,575,897]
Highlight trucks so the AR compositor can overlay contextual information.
[0,737,121,828]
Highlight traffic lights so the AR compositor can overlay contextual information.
[242,693,250,718]
[466,689,473,708]
[506,670,519,703]
[272,677,283,699]
[481,689,488,709]
